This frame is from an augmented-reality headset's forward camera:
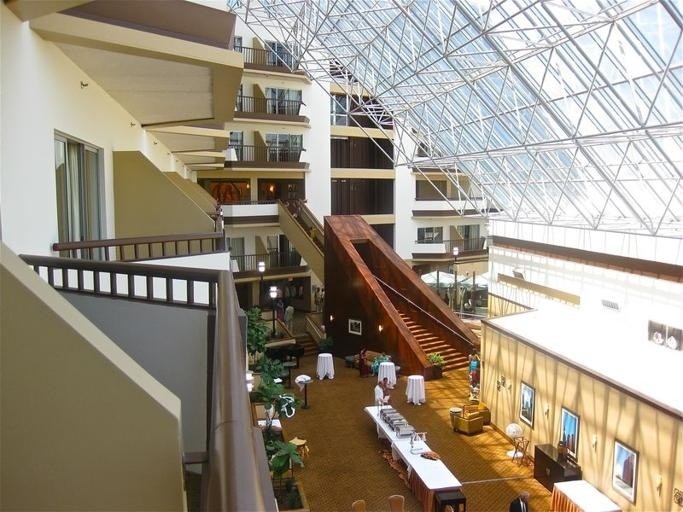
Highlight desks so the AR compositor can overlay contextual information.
[551,480,621,512]
[378,362,397,389]
[299,380,313,408]
[317,353,335,380]
[363,405,467,512]
[406,375,426,406]
[534,444,581,494]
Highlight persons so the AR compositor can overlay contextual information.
[314,287,325,312]
[374,381,385,406]
[285,302,294,333]
[310,224,318,242]
[468,348,481,387]
[359,348,389,378]
[382,377,389,405]
[509,492,530,512]
[282,195,303,219]
[276,299,285,322]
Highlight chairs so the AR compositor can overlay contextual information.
[352,500,366,512]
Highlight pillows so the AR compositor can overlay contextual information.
[467,412,479,419]
[478,402,486,410]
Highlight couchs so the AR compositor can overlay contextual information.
[453,416,483,435]
[464,406,491,424]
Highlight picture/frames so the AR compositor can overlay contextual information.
[519,380,535,429]
[613,438,638,506]
[348,317,363,335]
[560,406,579,462]
